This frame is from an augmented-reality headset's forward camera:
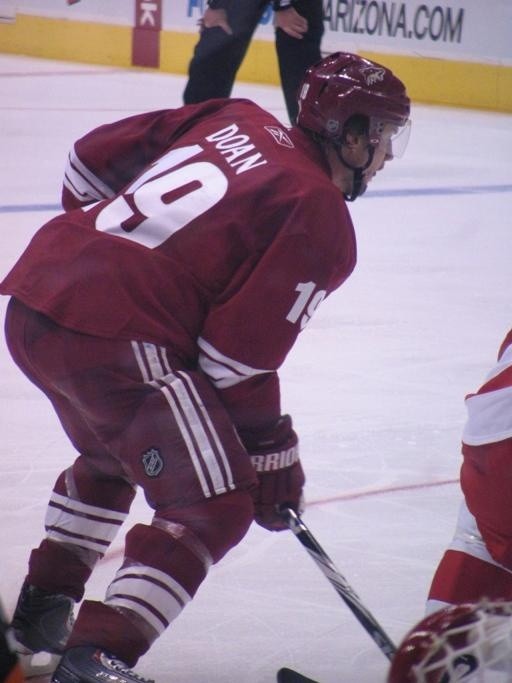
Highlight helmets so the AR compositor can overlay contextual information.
[392,600,512,680]
[295,52,414,160]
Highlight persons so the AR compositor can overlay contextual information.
[385,325,509,680]
[1,50,412,682]
[184,0,328,125]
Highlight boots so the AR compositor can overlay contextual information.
[51,646,152,682]
[14,577,77,653]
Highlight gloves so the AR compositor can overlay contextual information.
[238,416,304,531]
